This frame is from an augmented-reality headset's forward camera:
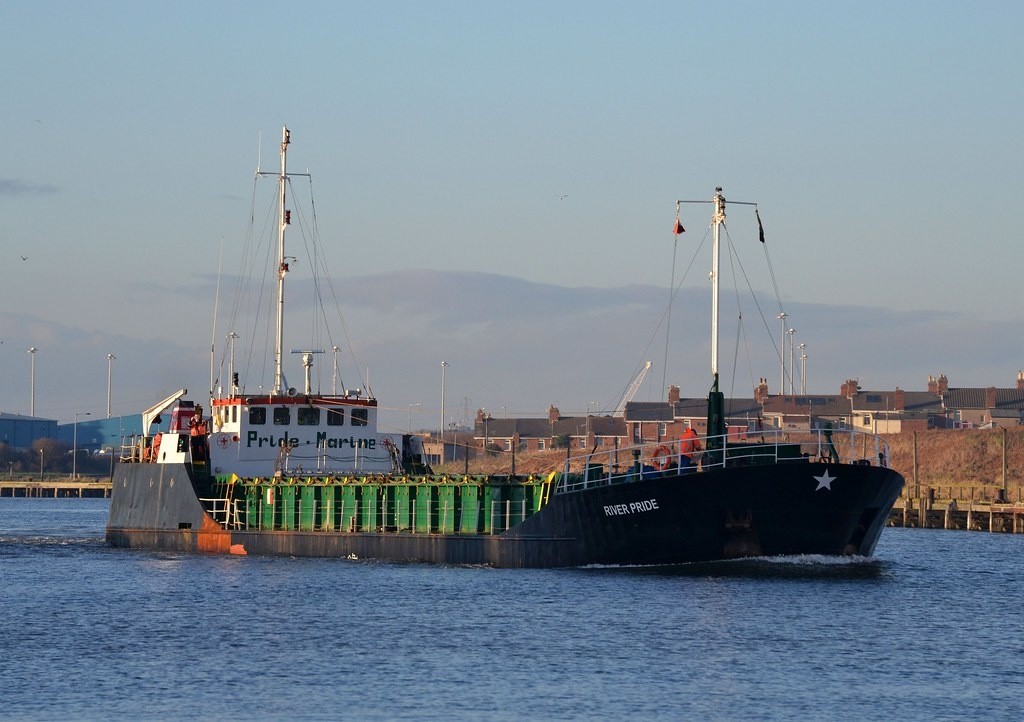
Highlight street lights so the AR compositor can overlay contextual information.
[73,412,90,481]
[996,424,1007,503]
[99,446,114,482]
[39,447,46,482]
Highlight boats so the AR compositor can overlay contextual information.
[104,126,907,566]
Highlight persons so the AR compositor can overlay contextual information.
[679,426,702,474]
[187,412,209,461]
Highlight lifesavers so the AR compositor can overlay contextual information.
[651,444,673,471]
